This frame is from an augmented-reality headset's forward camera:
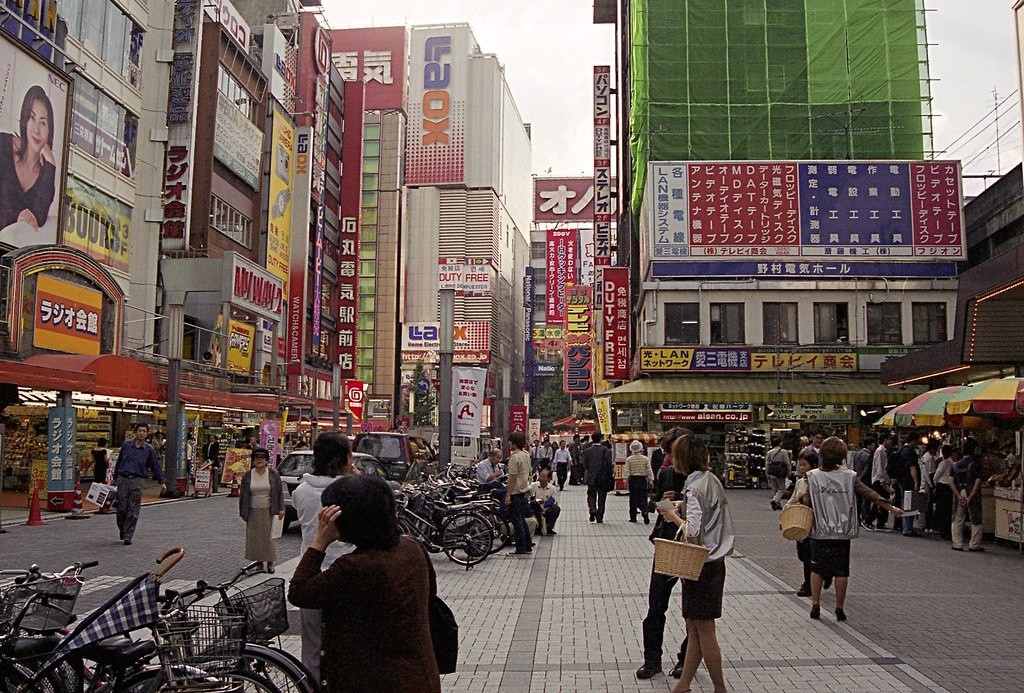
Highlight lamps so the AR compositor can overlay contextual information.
[221,310,237,315]
[248,370,258,374]
[277,97,303,104]
[259,372,269,376]
[230,364,234,370]
[289,111,314,118]
[277,359,300,365]
[899,384,907,390]
[236,368,247,372]
[230,315,249,321]
[246,320,260,326]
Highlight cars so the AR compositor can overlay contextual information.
[273,450,399,529]
[226,472,240,498]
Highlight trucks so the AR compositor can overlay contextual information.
[478,432,498,455]
[430,432,482,474]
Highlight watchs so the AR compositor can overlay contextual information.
[272,187,290,222]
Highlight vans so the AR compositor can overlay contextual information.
[350,431,435,486]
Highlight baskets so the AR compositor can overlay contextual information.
[2,576,83,631]
[214,575,289,644]
[779,501,814,542]
[154,603,248,681]
[652,520,710,581]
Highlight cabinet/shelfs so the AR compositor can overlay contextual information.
[154,409,199,479]
[76,411,117,483]
[204,425,233,460]
[0,415,50,493]
[710,431,725,467]
[993,487,1024,551]
[969,487,995,534]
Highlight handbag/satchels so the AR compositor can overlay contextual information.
[767,461,789,476]
[416,538,459,674]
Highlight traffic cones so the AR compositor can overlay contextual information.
[65,482,90,519]
[24,487,48,526]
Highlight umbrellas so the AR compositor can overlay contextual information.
[875,378,1024,429]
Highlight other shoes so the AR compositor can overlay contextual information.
[629,518,637,523]
[635,661,663,679]
[507,547,531,557]
[547,528,556,535]
[125,538,132,547]
[589,512,595,522]
[598,519,603,523]
[797,582,812,598]
[861,519,985,552]
[120,528,125,542]
[268,561,274,573]
[643,514,651,525]
[670,661,683,679]
[255,563,264,571]
[771,499,783,511]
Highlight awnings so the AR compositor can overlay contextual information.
[597,376,930,403]
[0,355,280,414]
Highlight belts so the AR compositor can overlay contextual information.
[122,473,140,479]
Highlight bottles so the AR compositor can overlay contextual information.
[1012,480,1015,490]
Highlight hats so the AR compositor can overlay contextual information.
[251,448,269,461]
[629,441,643,453]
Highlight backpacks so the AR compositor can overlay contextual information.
[886,448,913,479]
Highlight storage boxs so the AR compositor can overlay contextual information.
[904,490,919,511]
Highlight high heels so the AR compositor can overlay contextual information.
[810,603,820,619]
[835,608,847,623]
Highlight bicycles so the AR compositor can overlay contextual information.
[388,462,513,571]
[0,560,319,693]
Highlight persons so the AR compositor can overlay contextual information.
[531,434,611,490]
[530,469,561,536]
[759,425,983,622]
[581,433,612,523]
[637,425,734,693]
[202,435,220,471]
[90,437,109,484]
[503,433,532,556]
[623,440,654,524]
[0,85,56,248]
[287,475,441,693]
[110,422,166,545]
[247,437,261,450]
[238,448,286,574]
[292,432,359,685]
[475,448,506,500]
